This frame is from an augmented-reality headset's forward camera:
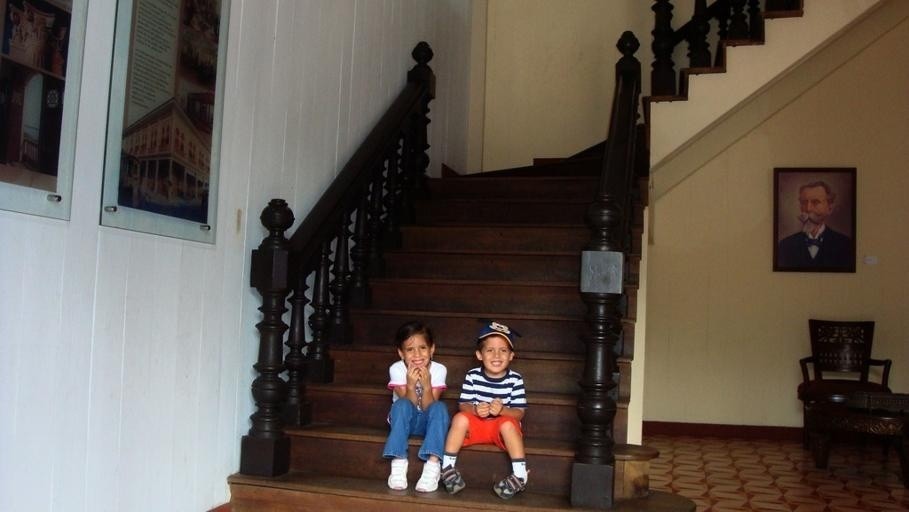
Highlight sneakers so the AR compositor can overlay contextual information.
[440,464,466,495]
[388,458,408,490]
[493,474,526,500]
[415,462,441,492]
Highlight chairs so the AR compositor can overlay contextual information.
[806,392,909,489]
[800,316,892,448]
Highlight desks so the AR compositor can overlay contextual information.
[798,379,891,401]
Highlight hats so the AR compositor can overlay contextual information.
[477,321,514,350]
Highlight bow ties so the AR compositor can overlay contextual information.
[804,239,821,247]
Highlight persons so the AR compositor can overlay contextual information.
[439,323,531,500]
[382,320,451,492]
[772,180,855,271]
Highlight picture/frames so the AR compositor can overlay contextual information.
[772,166,858,271]
